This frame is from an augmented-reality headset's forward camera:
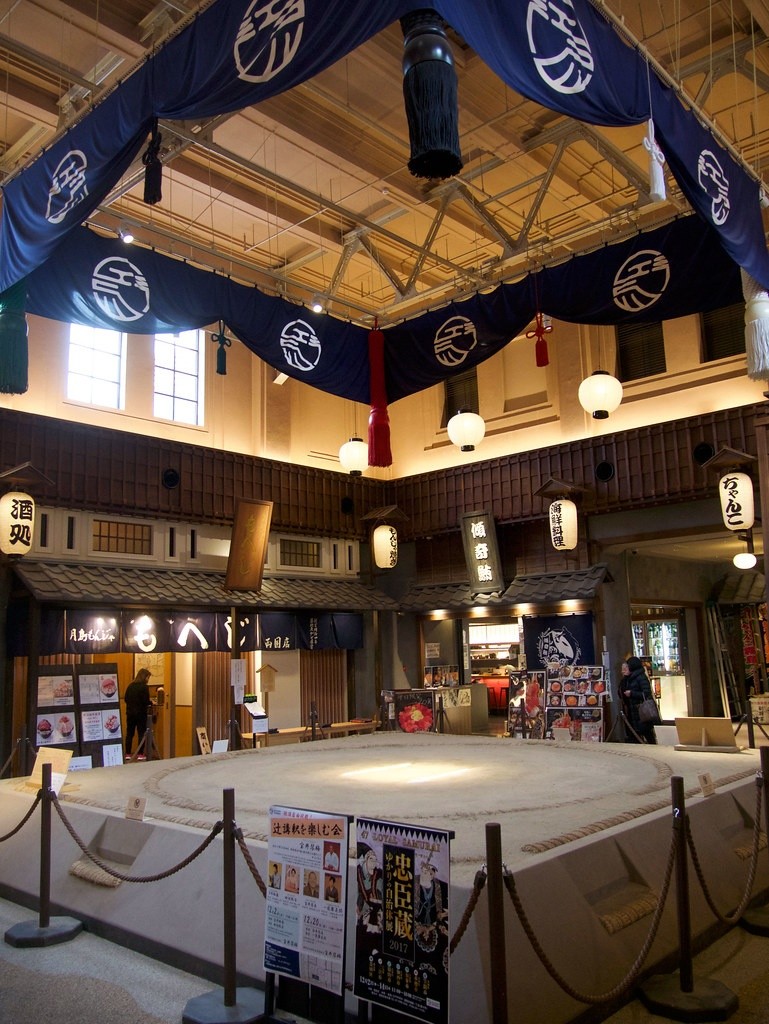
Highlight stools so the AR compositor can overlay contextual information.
[498,686,509,715]
[487,686,497,717]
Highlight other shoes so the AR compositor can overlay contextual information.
[125,754,131,759]
[137,753,146,760]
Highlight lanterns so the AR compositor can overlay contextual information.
[549,496,577,553]
[339,438,370,476]
[719,468,755,533]
[373,526,398,571]
[0,486,35,559]
[447,410,485,452]
[578,370,624,419]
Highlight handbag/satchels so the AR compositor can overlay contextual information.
[637,692,659,723]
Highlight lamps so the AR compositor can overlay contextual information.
[310,293,328,313]
[272,368,289,385]
[447,374,485,452]
[115,223,135,244]
[339,402,370,477]
[732,533,756,569]
[578,325,623,419]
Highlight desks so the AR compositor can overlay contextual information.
[241,721,382,748]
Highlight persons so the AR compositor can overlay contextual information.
[124,668,158,759]
[285,869,297,890]
[617,657,662,744]
[325,877,338,902]
[325,845,339,871]
[271,864,281,889]
[304,872,319,898]
